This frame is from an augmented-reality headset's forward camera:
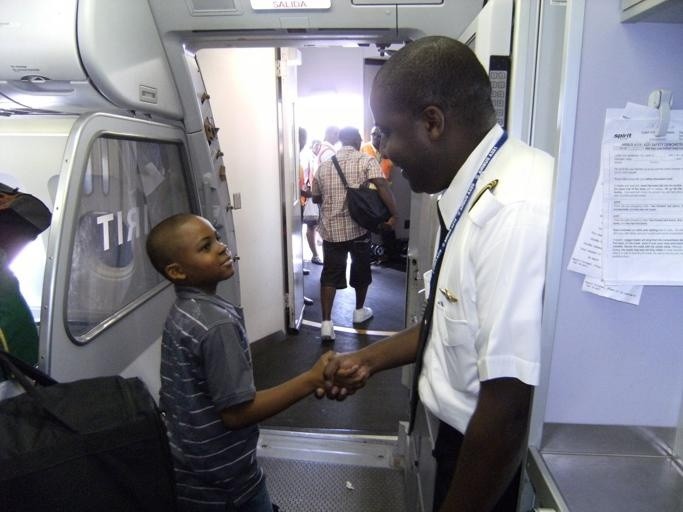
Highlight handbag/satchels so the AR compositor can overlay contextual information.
[347,188,390,233]
[0,182,52,264]
[0,350,178,511]
[301,184,312,198]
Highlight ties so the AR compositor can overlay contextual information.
[407,202,447,434]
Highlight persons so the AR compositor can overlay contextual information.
[1,260,42,401]
[145,212,367,511]
[299,124,400,343]
[314,33,556,511]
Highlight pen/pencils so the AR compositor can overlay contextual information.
[469,179,498,213]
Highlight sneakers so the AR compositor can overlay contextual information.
[320,320,336,341]
[353,306,373,323]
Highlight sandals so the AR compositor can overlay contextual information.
[311,256,324,265]
